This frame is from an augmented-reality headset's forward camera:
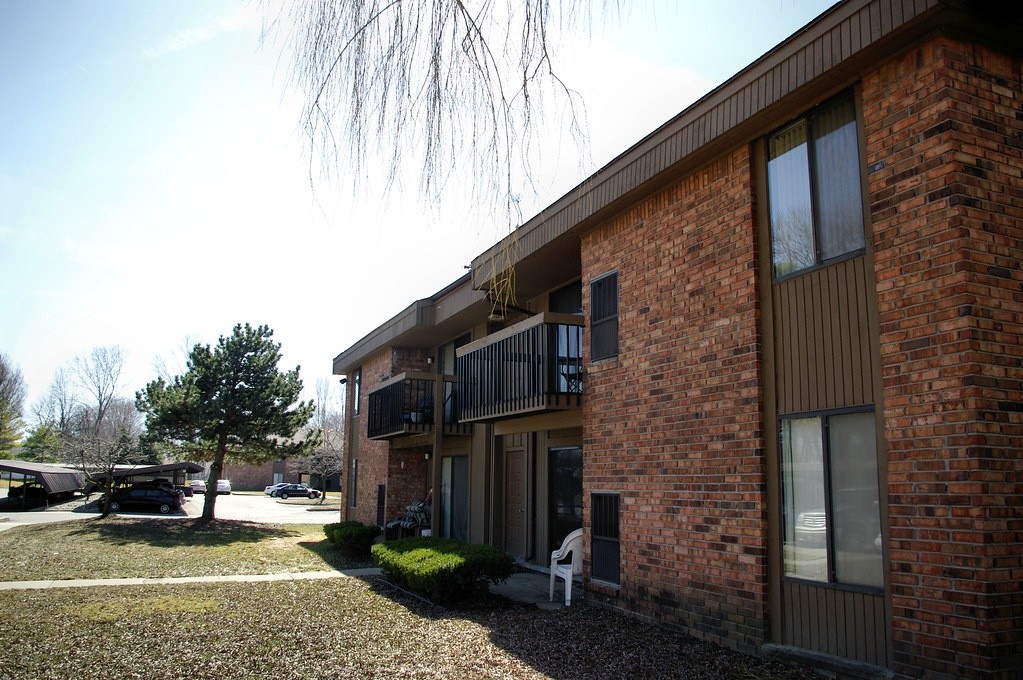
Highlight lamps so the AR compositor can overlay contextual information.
[427,356,434,368]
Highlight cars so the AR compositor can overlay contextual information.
[275,484,322,499]
[98,479,185,514]
[216,480,232,495]
[8,483,62,503]
[190,480,207,494]
[264,482,291,497]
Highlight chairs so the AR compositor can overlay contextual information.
[549,527,583,606]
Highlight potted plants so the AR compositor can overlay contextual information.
[410,407,424,422]
